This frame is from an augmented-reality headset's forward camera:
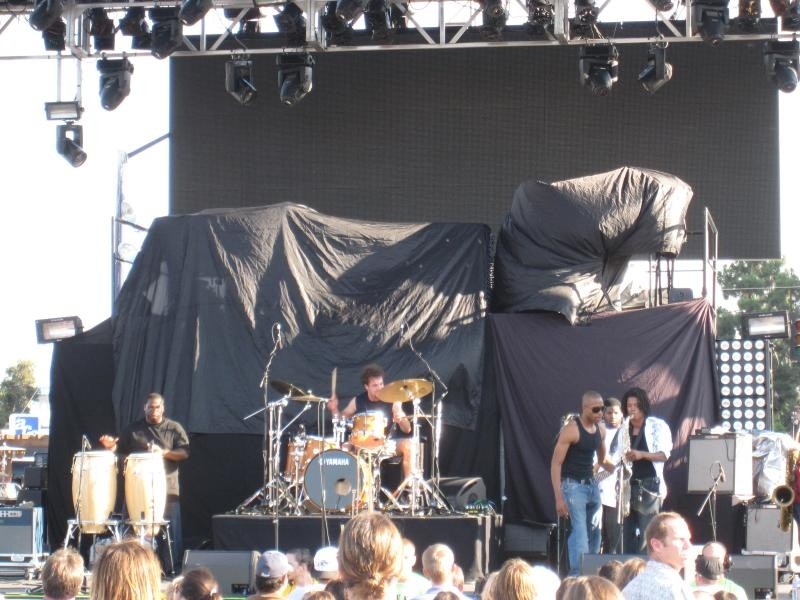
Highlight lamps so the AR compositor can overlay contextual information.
[35,316,82,343]
[742,309,789,433]
[28,0,800,168]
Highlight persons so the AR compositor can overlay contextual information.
[243,545,351,600]
[41,546,83,600]
[390,536,748,600]
[608,387,674,554]
[166,567,223,600]
[336,508,404,600]
[620,511,697,600]
[89,536,163,600]
[328,362,418,489]
[550,389,615,578]
[100,393,189,579]
[590,397,626,556]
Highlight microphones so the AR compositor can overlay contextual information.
[719,464,726,482]
[83,435,92,450]
[276,324,283,349]
[434,391,447,408]
[398,323,404,348]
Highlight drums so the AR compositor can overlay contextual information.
[72,449,119,535]
[349,409,386,448]
[282,433,339,484]
[123,451,168,538]
[302,449,371,510]
[359,438,398,458]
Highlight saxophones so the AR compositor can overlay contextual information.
[613,413,636,519]
[771,428,800,532]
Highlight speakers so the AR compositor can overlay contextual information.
[580,553,647,577]
[685,434,735,494]
[726,554,775,600]
[440,476,486,511]
[182,549,260,597]
[0,507,44,575]
[741,503,793,552]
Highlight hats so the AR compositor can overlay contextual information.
[255,550,294,578]
[312,546,338,578]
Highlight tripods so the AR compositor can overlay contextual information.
[228,341,456,516]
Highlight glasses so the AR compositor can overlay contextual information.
[592,405,605,414]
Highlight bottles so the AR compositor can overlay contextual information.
[792,575,800,600]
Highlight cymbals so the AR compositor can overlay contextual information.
[376,378,433,403]
[287,395,331,403]
[268,377,308,398]
[403,414,437,420]
